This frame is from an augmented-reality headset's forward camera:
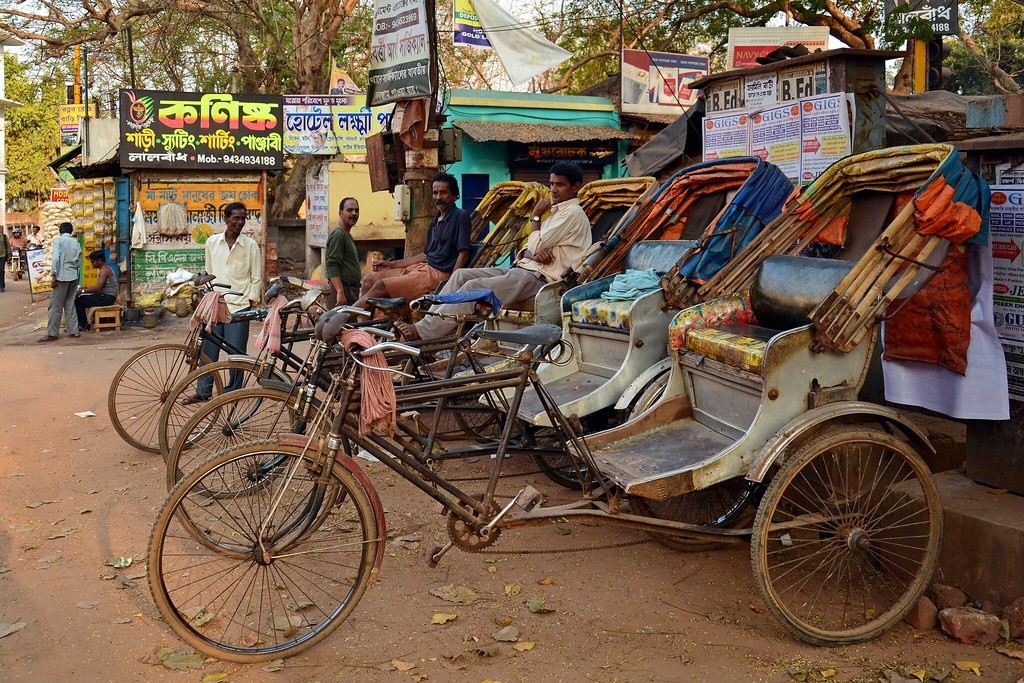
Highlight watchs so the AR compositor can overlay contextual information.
[530,216,541,222]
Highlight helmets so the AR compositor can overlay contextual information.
[12,228,22,239]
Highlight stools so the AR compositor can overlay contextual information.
[92,307,123,333]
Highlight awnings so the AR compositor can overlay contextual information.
[46,144,82,170]
[451,120,641,143]
[66,153,120,179]
[621,112,681,124]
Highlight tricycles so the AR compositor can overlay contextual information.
[146,140,993,665]
[107,157,794,562]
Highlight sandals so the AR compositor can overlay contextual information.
[37,335,57,342]
[72,332,81,338]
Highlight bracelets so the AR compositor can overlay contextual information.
[51,281,55,283]
[82,289,84,294]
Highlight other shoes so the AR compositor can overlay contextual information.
[0,288,5,292]
[309,306,324,321]
[182,395,208,404]
[78,324,90,331]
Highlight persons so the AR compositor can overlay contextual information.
[37,222,82,342]
[182,202,262,405]
[74,250,118,332]
[347,172,471,322]
[324,197,362,346]
[331,79,345,94]
[393,161,592,342]
[0,226,45,292]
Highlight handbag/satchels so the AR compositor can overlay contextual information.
[158,197,187,236]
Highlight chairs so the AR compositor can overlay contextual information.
[561,240,704,378]
[411,241,484,309]
[675,254,864,433]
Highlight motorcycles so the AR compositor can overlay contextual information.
[7,238,45,281]
